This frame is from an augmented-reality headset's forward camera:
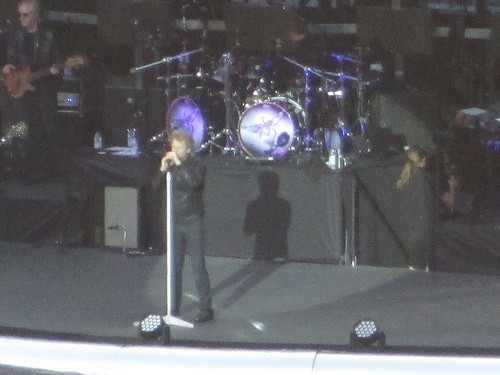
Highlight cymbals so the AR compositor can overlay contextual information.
[96,0,175,47]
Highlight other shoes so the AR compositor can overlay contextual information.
[195,311,213,322]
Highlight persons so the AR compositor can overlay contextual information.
[2,0,61,167]
[396,146,459,272]
[161,130,215,323]
[262,24,310,83]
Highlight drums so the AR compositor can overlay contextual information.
[287,88,314,123]
[235,56,274,93]
[327,88,358,126]
[166,94,240,155]
[236,96,308,161]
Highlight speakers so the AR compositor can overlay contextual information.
[102,184,141,251]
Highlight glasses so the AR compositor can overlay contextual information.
[19,10,35,18]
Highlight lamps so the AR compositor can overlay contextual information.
[351,320,388,350]
[138,313,171,344]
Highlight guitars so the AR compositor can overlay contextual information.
[3,52,90,100]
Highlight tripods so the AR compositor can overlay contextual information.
[194,52,240,154]
[308,54,373,156]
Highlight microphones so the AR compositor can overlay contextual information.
[165,159,172,163]
